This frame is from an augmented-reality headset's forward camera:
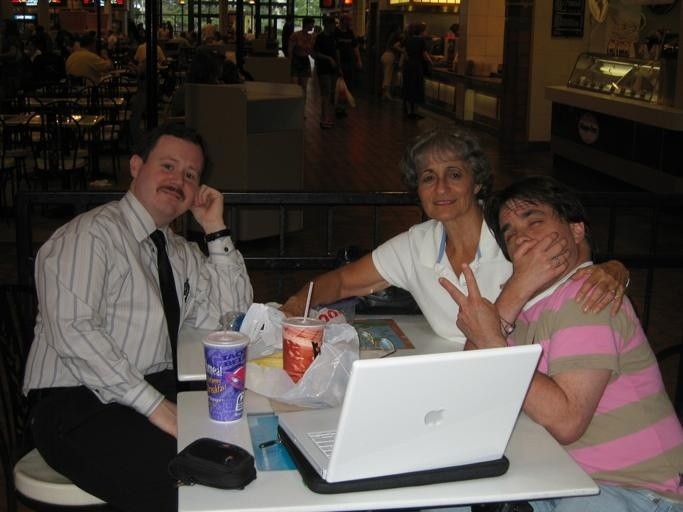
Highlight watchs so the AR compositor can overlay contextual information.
[204,227,233,242]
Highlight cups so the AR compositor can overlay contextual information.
[201,330,248,423]
[279,317,326,384]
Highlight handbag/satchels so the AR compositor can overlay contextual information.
[398,51,406,72]
[170,437,258,487]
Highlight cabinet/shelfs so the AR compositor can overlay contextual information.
[566,52,660,103]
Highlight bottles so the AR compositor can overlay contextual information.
[217,311,264,332]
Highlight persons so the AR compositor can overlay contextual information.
[437,171,682,510]
[1,15,461,129]
[21,122,253,512]
[272,120,632,349]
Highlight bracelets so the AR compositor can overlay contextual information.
[497,315,516,337]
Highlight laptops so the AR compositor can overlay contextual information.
[278,344,543,483]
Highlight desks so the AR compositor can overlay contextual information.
[172,315,599,512]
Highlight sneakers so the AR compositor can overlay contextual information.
[320,119,336,128]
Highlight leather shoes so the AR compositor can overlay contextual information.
[408,111,427,119]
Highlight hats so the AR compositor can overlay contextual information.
[322,16,338,24]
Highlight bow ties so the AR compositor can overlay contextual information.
[148,226,181,379]
